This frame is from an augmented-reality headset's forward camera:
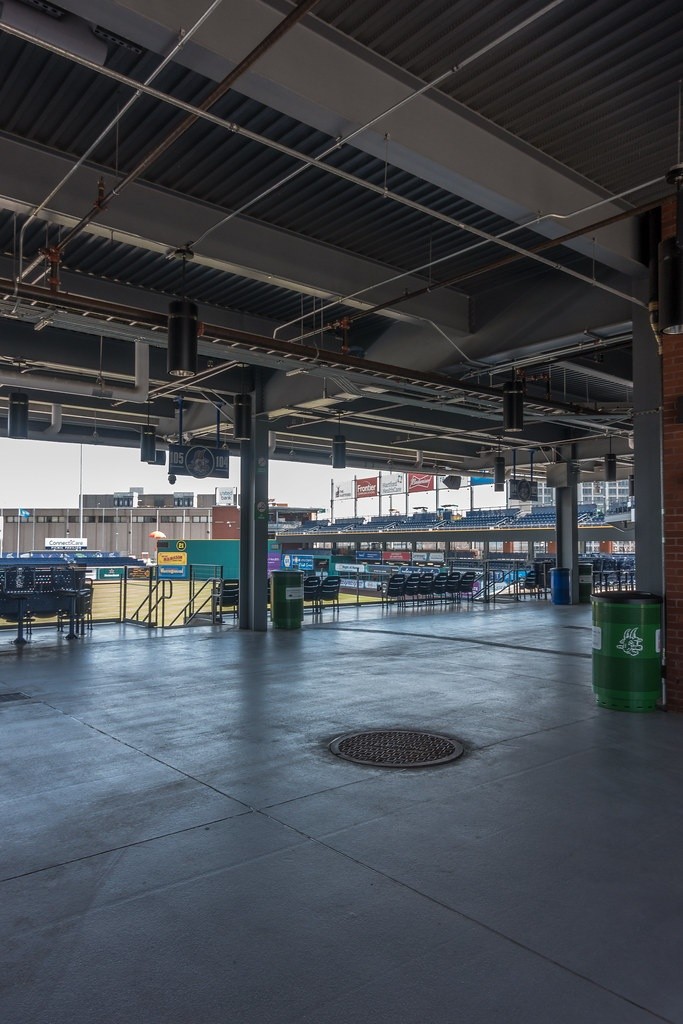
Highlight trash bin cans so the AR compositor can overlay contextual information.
[590,590,663,712]
[270,570,305,629]
[550,568,570,605]
[578,563,594,603]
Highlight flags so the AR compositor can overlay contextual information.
[20,508,31,519]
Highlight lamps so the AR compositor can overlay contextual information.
[628,475,635,496]
[233,362,252,440]
[267,578,270,604]
[332,411,346,469]
[604,434,616,482]
[494,434,505,491]
[148,451,166,465]
[443,475,461,489]
[167,249,198,377]
[503,360,523,433]
[658,168,683,335]
[8,358,29,440]
[140,399,156,462]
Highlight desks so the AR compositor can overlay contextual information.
[0,569,92,635]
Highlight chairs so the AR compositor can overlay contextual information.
[519,556,635,600]
[211,578,239,620]
[447,511,605,526]
[304,576,341,615]
[381,571,475,608]
[0,565,95,647]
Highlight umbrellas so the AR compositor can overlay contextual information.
[149,531,166,550]
